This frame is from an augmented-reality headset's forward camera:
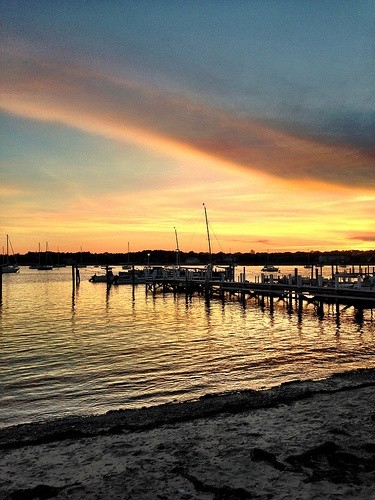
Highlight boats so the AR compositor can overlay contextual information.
[1,264,375,292]
